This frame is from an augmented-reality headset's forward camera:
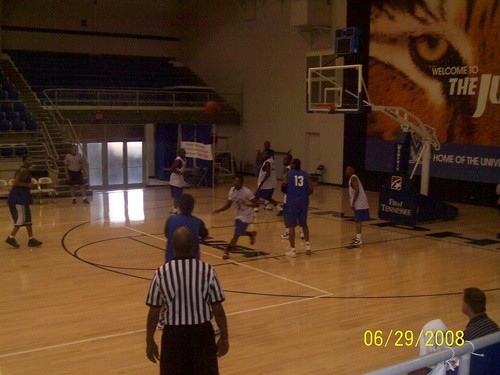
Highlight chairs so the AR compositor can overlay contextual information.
[0,177,57,200]
[0,71,38,157]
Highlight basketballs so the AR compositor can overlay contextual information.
[205,101,221,116]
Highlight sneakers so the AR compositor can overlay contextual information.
[348,237,363,246]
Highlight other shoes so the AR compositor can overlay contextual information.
[305,242,311,254]
[71,198,77,204]
[169,208,178,216]
[222,250,230,260]
[5,236,20,248]
[263,204,273,210]
[249,230,258,245]
[279,232,290,239]
[83,198,90,204]
[299,232,304,238]
[254,207,259,212]
[28,238,43,246]
[285,250,297,258]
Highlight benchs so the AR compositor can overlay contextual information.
[1,50,207,97]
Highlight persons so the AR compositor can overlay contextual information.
[211,172,259,260]
[169,149,187,215]
[459,287,500,375]
[155,193,220,336]
[64,142,90,204]
[253,140,283,215]
[346,165,369,246]
[408,319,460,375]
[281,155,305,240]
[6,157,43,247]
[281,159,314,257]
[145,227,229,375]
